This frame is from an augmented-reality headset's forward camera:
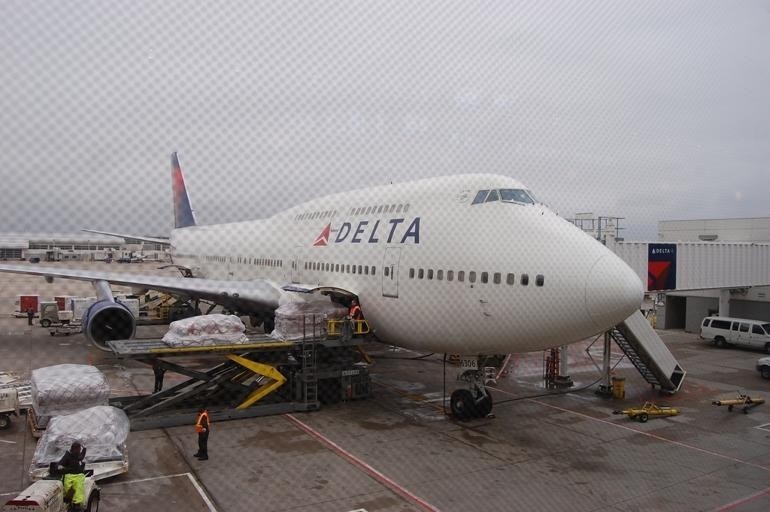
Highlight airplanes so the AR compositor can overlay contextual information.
[1,150,645,419]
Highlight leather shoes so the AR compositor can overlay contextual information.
[194,453,208,460]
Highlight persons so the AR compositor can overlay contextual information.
[57,441,87,473]
[347,299,360,321]
[192,405,211,460]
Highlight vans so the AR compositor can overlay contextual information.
[700,316,769,353]
